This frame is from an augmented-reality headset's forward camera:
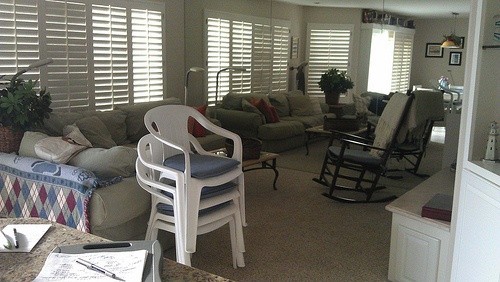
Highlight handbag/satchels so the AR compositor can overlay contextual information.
[35,123,93,164]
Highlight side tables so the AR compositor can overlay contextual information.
[320,101,348,114]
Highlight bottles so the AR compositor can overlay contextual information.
[438,76,448,90]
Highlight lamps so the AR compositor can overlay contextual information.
[10,57,53,83]
[214,67,246,118]
[440,13,461,48]
[184,66,205,106]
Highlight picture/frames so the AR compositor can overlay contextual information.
[448,51,462,65]
[425,42,443,58]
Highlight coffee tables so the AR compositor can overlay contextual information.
[306,124,375,156]
[242,151,281,191]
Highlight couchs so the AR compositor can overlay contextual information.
[210,88,326,153]
[0,99,227,251]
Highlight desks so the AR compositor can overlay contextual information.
[0,216,236,282]
[383,169,456,282]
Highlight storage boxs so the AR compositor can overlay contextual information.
[421,192,452,221]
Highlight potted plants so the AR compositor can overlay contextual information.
[318,67,355,105]
[0,78,54,155]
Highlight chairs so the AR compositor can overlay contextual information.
[362,90,444,179]
[311,92,414,204]
[135,105,247,270]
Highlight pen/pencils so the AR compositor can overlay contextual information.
[0,230,12,249]
[76,258,115,278]
[13,227,18,248]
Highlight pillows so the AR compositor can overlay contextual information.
[242,96,282,124]
[76,116,116,150]
[193,105,208,137]
